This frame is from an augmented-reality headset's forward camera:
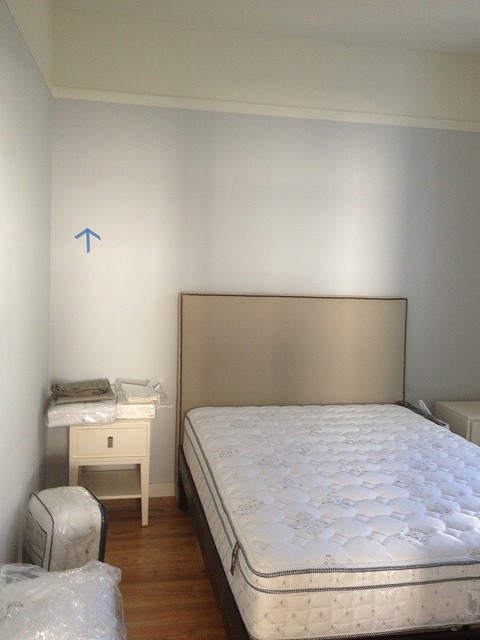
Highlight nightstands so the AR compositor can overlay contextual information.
[67,418,153,527]
[434,400,480,446]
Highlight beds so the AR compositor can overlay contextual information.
[173,289,480,640]
